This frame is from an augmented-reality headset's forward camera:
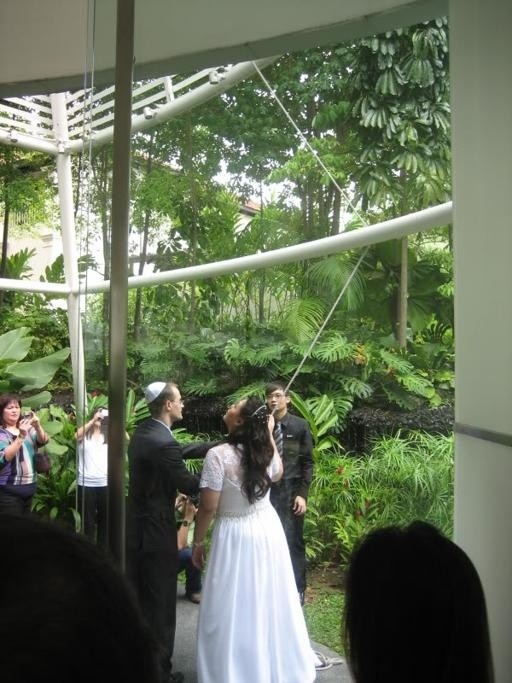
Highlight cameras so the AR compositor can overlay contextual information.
[100,409,109,417]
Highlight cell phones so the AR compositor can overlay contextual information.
[23,411,32,423]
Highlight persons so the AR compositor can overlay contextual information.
[0,505,164,683]
[189,395,319,683]
[0,390,52,513]
[125,380,231,683]
[263,381,315,609]
[172,490,204,604]
[72,404,131,546]
[340,519,496,683]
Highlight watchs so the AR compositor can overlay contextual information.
[181,519,192,526]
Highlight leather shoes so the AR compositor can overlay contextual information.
[185,589,200,603]
[170,672,183,682]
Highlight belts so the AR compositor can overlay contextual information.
[273,478,302,488]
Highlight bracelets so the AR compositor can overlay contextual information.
[18,433,26,440]
[191,538,205,547]
[89,420,95,426]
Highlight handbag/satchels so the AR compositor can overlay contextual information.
[33,443,52,473]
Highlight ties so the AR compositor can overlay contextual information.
[274,421,283,459]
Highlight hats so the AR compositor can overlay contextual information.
[144,381,167,403]
